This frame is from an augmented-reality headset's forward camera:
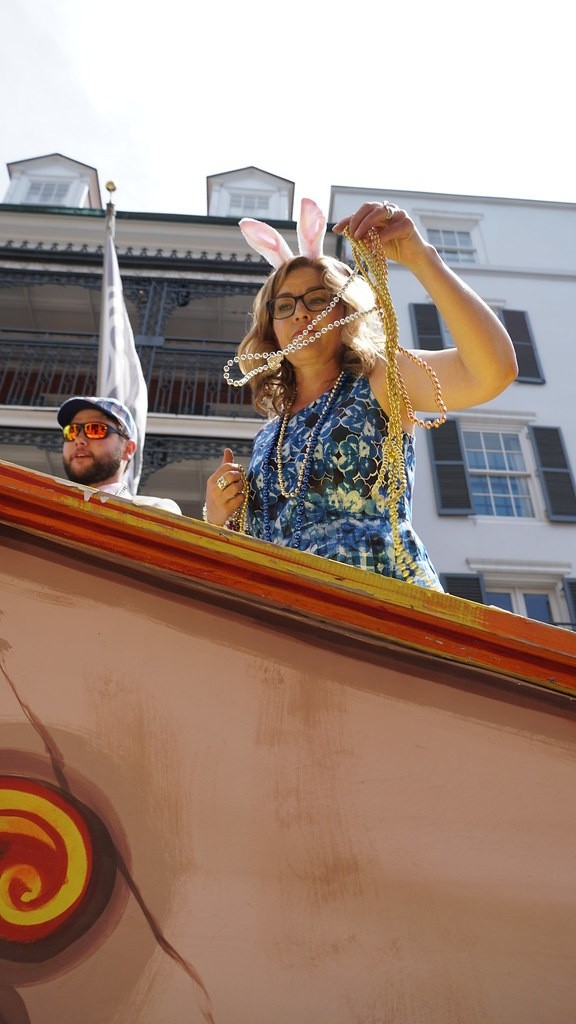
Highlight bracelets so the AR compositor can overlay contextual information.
[201,500,237,529]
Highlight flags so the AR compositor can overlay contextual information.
[96,205,149,494]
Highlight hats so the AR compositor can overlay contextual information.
[57,396,137,444]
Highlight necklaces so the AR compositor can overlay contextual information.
[223,223,446,593]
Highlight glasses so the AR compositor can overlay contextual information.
[265,286,337,320]
[62,422,129,442]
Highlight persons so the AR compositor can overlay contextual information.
[198,201,520,597]
[56,397,183,517]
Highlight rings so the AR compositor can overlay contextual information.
[383,200,394,221]
[216,476,228,490]
